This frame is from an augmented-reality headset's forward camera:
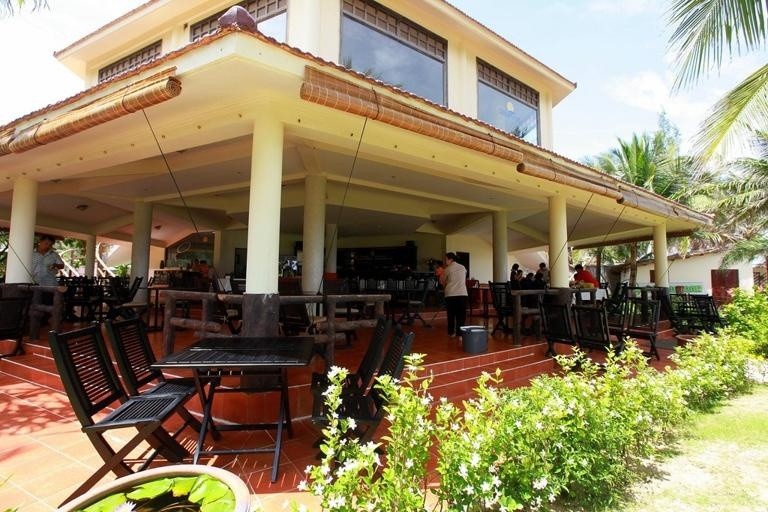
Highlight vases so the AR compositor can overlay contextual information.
[429,265,434,271]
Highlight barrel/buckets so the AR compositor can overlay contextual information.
[460,324,489,354]
[675,334,697,346]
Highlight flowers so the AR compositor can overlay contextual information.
[115,501,137,512]
[423,256,437,265]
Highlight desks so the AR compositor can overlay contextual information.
[150,336,316,484]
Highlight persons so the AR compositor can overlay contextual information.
[434,260,446,290]
[440,251,470,339]
[509,262,551,308]
[193,260,209,292]
[31,235,65,329]
[192,259,200,272]
[573,264,600,289]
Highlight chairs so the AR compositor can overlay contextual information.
[395,281,447,328]
[488,279,724,372]
[48,313,208,508]
[311,314,415,474]
[57,275,144,328]
